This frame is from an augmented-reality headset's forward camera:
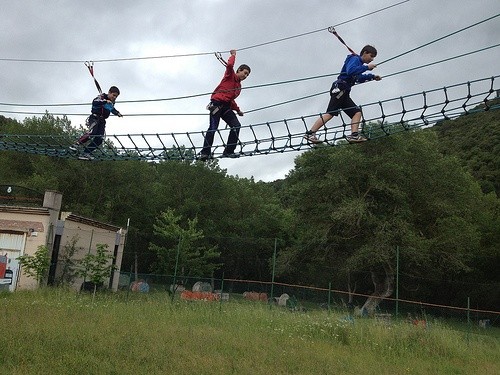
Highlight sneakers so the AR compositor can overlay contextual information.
[347,133,367,143]
[303,130,322,143]
[76,153,95,161]
[223,151,240,158]
[199,151,214,159]
[69,144,78,152]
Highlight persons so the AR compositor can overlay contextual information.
[70,86,123,160]
[305,45,383,142]
[199,49,251,157]
